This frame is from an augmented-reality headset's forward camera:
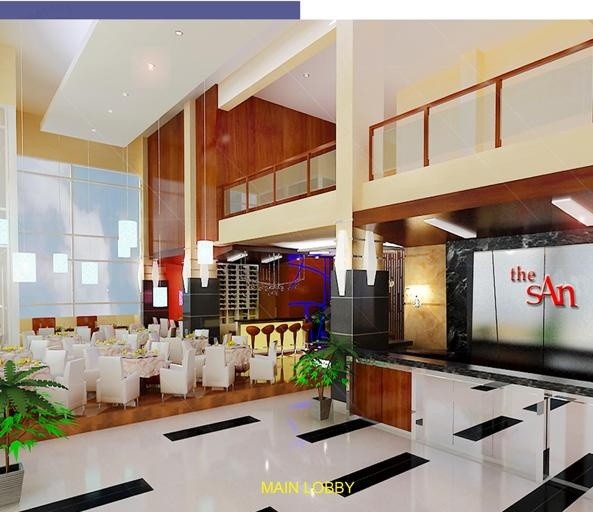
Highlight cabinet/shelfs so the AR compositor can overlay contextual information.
[216,263,259,342]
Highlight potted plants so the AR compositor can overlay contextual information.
[0,359,81,507]
[290,327,360,421]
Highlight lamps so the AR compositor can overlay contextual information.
[53,134,68,273]
[153,118,168,307]
[261,253,283,264]
[227,252,248,262]
[118,148,131,257]
[196,79,213,265]
[118,142,138,249]
[12,47,37,283]
[81,136,98,284]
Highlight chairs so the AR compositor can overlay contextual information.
[21,317,278,419]
[289,323,301,357]
[302,322,313,348]
[275,324,288,359]
[0,329,251,396]
